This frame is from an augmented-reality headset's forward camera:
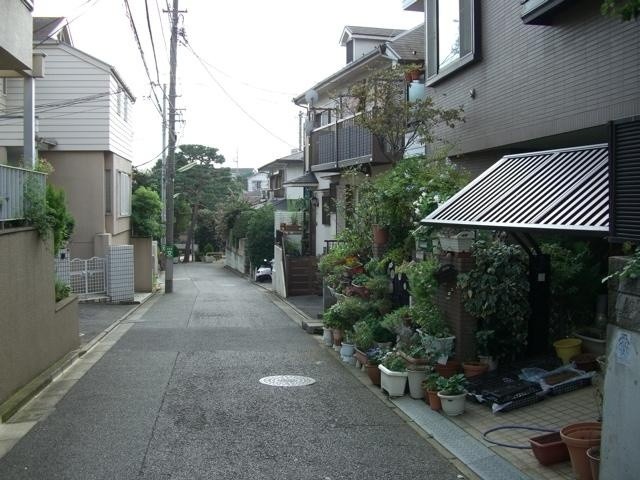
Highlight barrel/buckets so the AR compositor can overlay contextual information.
[554,338,581,364]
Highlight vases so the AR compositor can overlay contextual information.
[528,421,602,480]
[461,362,489,379]
[551,327,608,370]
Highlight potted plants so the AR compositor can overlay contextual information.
[365,190,395,244]
[318,245,469,418]
[286,215,301,232]
[401,62,423,82]
[475,329,500,373]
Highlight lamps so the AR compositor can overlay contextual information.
[310,193,319,207]
